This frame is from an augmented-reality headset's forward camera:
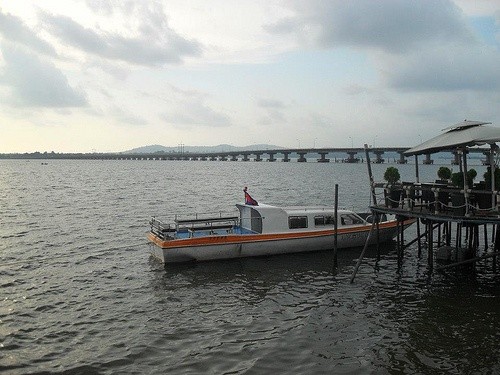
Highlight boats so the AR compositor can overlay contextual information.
[147,189,417,267]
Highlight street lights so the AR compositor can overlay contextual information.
[343,132,424,150]
